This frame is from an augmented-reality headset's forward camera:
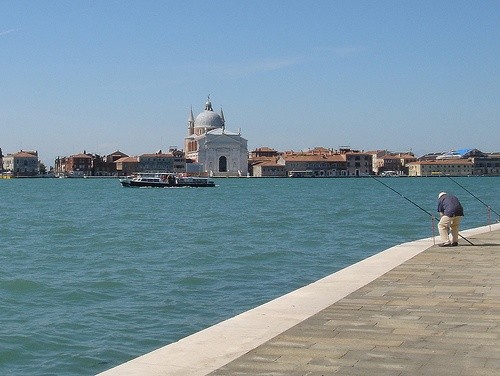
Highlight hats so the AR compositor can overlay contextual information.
[438,192,447,199]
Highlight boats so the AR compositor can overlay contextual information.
[121,171,216,187]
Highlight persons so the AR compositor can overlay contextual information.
[437,191,464,247]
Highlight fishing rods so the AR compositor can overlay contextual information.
[423,159,500,223]
[370,176,474,246]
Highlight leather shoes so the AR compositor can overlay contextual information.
[452,242,459,247]
[438,243,452,248]
